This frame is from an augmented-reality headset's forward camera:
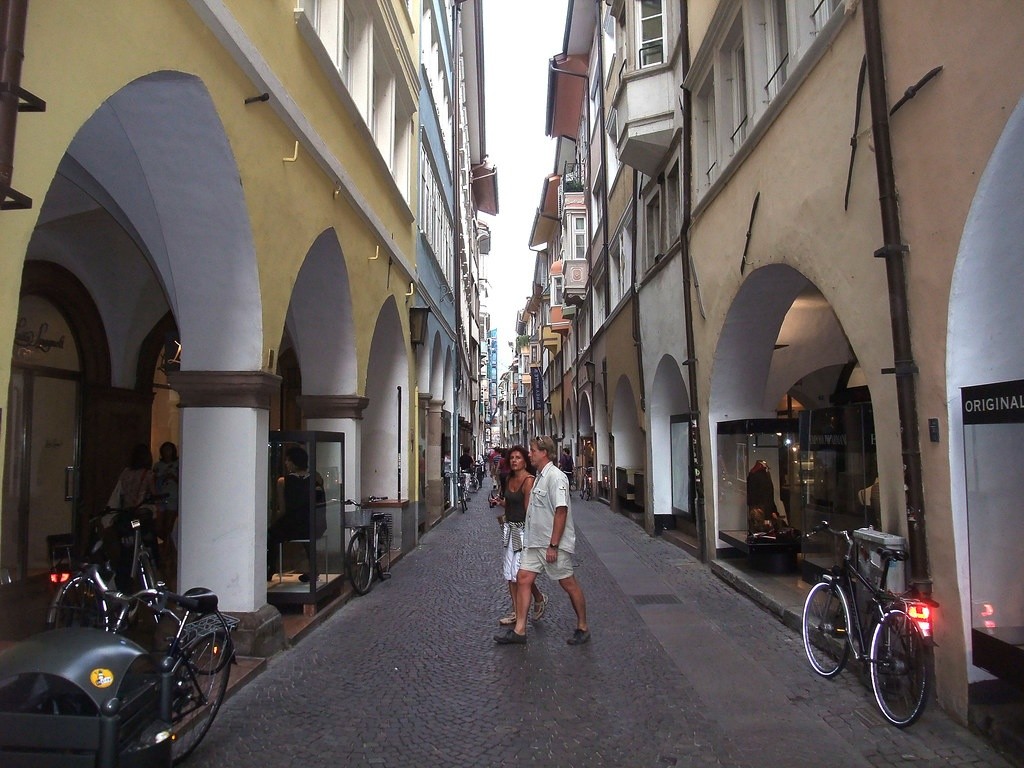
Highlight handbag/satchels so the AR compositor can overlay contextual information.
[106,467,128,509]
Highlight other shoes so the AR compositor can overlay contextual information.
[298,572,320,583]
[499,611,516,624]
[533,593,548,621]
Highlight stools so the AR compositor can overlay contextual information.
[276,535,328,592]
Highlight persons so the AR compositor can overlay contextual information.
[559,448,574,484]
[493,435,590,644]
[488,445,549,625]
[459,446,473,496]
[747,459,779,525]
[488,446,513,500]
[267,446,326,590]
[100,441,179,587]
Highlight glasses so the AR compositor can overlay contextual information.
[536,436,544,442]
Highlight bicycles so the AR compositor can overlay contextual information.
[579,466,594,501]
[458,468,479,513]
[802,520,939,728]
[46,508,241,767]
[345,496,391,596]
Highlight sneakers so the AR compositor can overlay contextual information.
[567,629,591,645]
[493,630,527,644]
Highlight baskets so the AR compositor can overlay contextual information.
[344,509,373,527]
[453,475,466,484]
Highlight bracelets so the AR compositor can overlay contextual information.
[550,544,558,549]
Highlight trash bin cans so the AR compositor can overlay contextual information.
[0,626,176,768]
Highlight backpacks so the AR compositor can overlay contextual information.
[563,454,572,472]
